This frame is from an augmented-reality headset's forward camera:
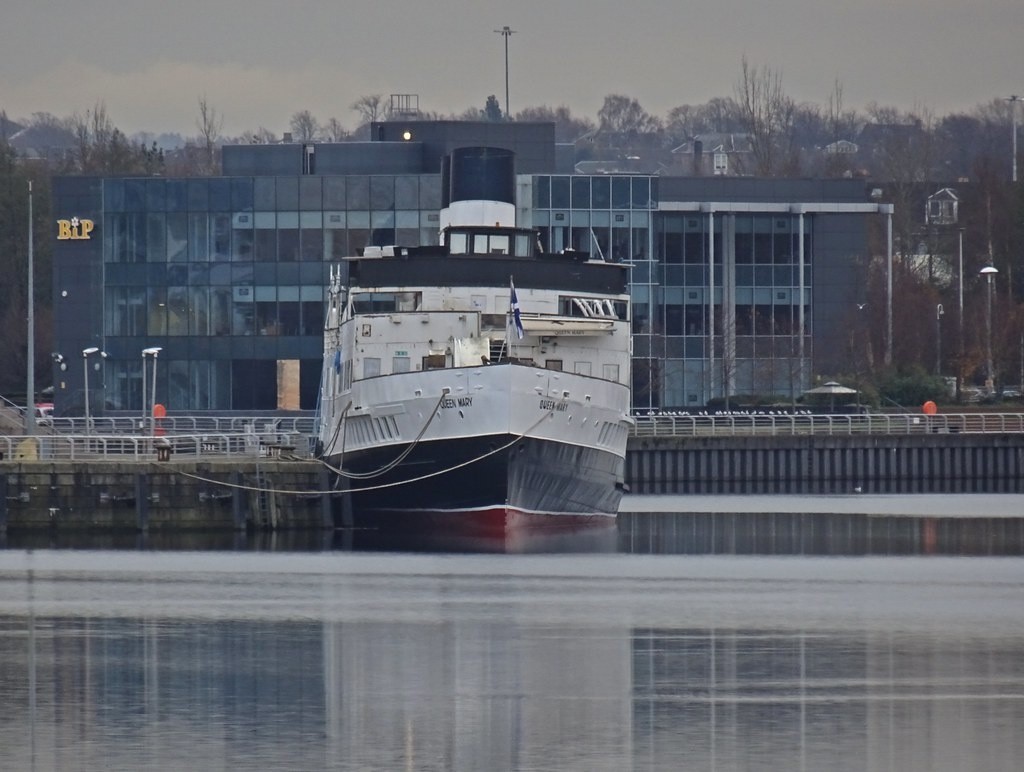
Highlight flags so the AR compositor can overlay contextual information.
[511,279,524,339]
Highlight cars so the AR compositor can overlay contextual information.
[1002,388,1021,400]
[34,407,53,426]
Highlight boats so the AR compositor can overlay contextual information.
[311,146,633,552]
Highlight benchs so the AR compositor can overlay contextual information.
[933,425,959,433]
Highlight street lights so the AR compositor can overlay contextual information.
[937,304,945,374]
[980,268,998,403]
[493,26,517,117]
[142,347,164,454]
[83,348,100,452]
[956,226,966,403]
